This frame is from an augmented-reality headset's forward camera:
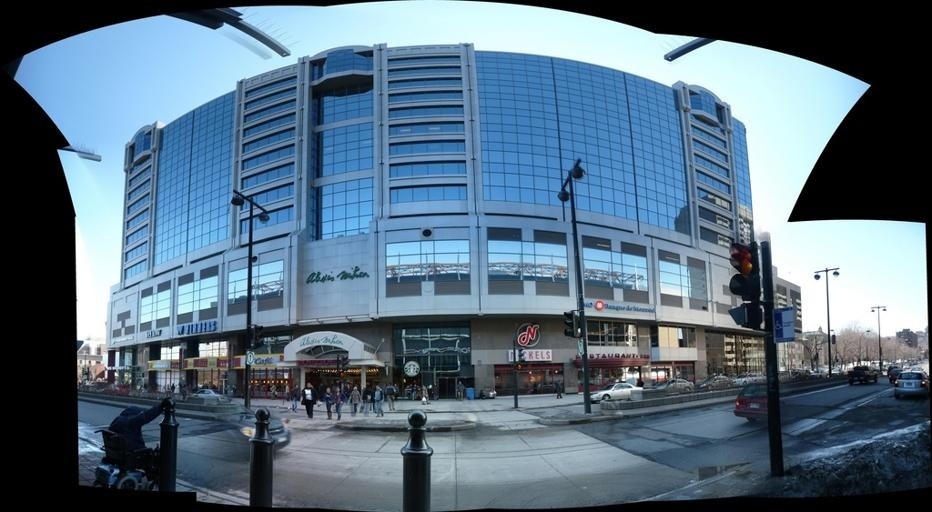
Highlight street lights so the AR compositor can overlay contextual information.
[871,305,887,372]
[232,188,269,408]
[859,329,871,365]
[557,158,591,414]
[814,267,840,378]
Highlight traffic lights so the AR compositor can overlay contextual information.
[562,310,574,337]
[518,347,526,370]
[728,241,760,329]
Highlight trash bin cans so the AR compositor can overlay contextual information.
[465,388,474,400]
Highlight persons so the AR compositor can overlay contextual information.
[556,380,563,398]
[109,397,176,466]
[457,381,466,401]
[269,379,430,419]
[636,378,644,386]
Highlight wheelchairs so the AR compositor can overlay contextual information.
[94,428,161,490]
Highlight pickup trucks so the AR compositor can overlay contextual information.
[848,365,878,384]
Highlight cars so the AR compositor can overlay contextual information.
[590,382,643,403]
[656,378,695,394]
[698,375,736,391]
[179,398,290,459]
[732,383,769,422]
[888,365,929,398]
[779,369,810,381]
[736,373,766,385]
[191,389,231,403]
